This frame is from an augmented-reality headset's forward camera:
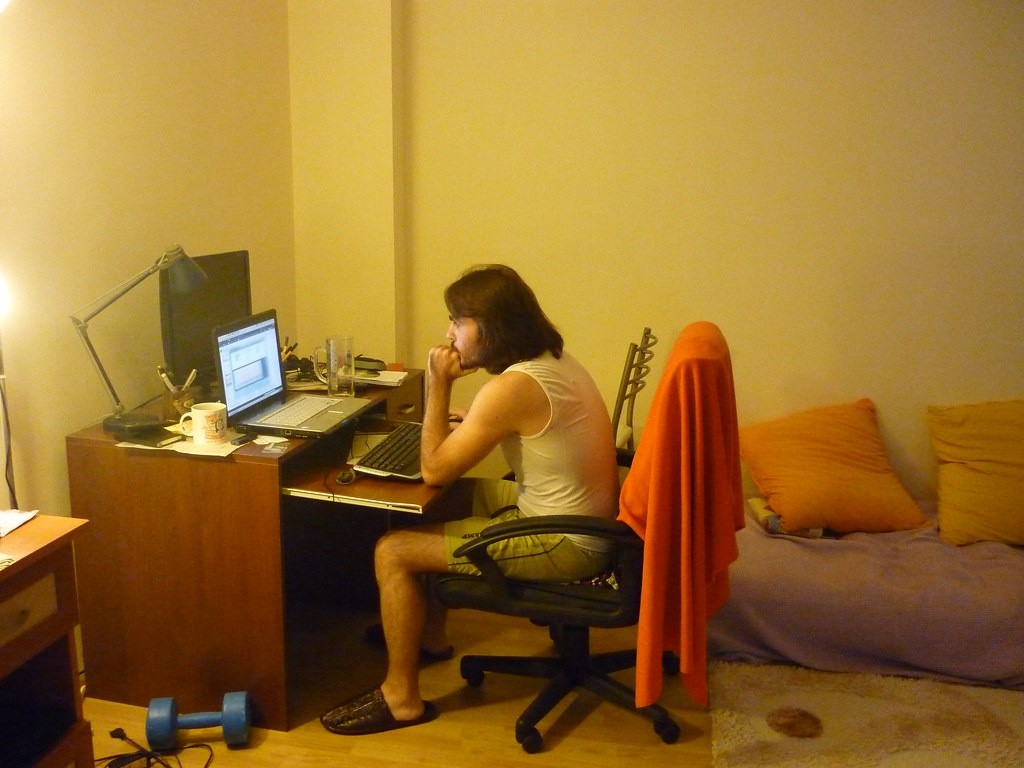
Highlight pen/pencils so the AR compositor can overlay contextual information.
[280,336,298,362]
[181,368,197,392]
[157,365,175,392]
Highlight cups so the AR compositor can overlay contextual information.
[179,402,226,443]
[312,335,356,398]
[165,386,194,426]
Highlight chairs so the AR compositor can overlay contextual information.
[420,319,741,754]
[613,327,657,483]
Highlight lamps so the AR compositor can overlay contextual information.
[72,242,209,446]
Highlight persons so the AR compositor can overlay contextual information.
[319,266,621,735]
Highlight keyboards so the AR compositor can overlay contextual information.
[352,422,424,483]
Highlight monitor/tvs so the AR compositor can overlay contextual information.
[159,250,252,388]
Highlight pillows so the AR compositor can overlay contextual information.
[726,399,930,533]
[925,398,1023,546]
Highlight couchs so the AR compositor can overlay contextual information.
[704,503,1024,690]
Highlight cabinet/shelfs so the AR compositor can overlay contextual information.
[0,512,97,768]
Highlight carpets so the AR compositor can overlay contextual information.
[706,657,1023,768]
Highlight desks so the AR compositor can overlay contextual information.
[64,364,430,733]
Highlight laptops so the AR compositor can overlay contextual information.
[211,309,372,439]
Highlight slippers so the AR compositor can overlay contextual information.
[320,694,437,735]
[366,622,455,662]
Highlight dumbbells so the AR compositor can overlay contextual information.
[141,688,255,754]
